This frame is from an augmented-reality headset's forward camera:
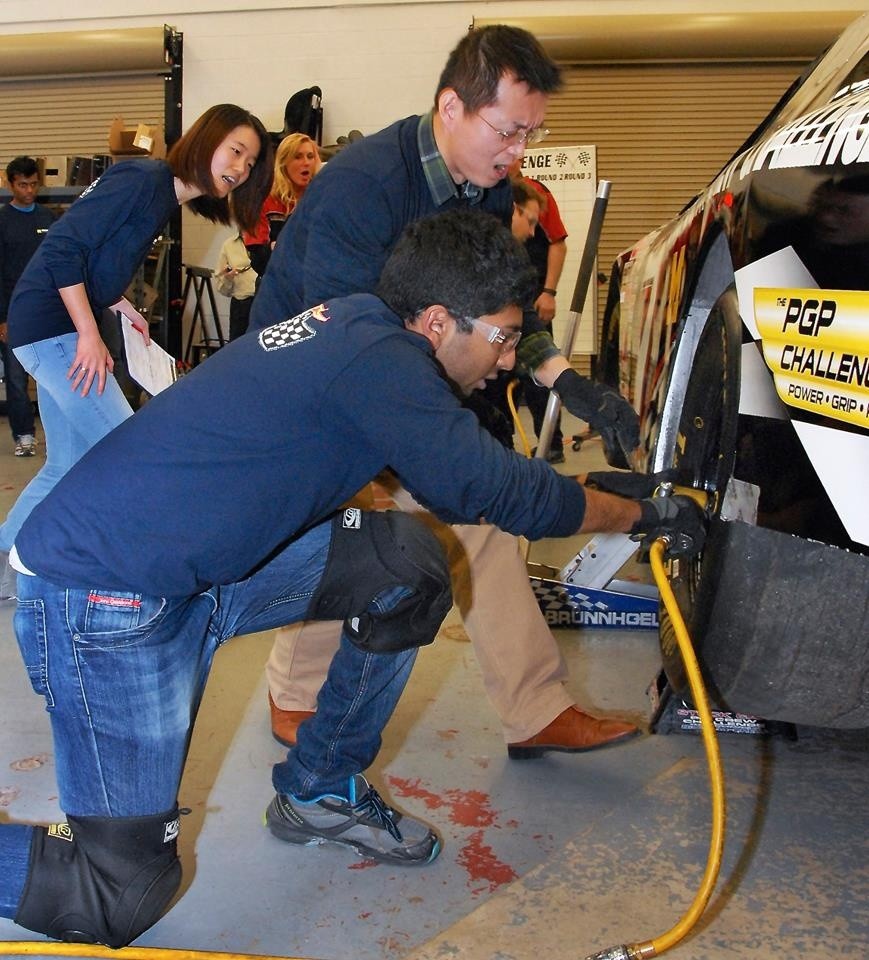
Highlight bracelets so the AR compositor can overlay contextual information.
[542,287,556,297]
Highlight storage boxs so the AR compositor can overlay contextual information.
[109,116,167,164]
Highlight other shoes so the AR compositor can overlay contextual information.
[530,447,564,464]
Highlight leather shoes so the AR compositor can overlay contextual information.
[269,691,316,747]
[507,705,637,759]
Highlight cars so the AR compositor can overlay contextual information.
[586,72,869,732]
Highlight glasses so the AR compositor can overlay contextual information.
[415,309,522,354]
[457,96,552,146]
[517,206,538,226]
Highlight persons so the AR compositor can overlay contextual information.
[215,228,257,341]
[506,160,568,463]
[0,203,710,932]
[0,155,57,457]
[0,104,274,591]
[242,133,323,288]
[264,25,644,760]
[510,183,545,244]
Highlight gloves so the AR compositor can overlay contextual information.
[624,494,705,555]
[585,469,676,500]
[554,369,639,454]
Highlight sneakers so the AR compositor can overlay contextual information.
[14,434,37,456]
[265,772,442,866]
